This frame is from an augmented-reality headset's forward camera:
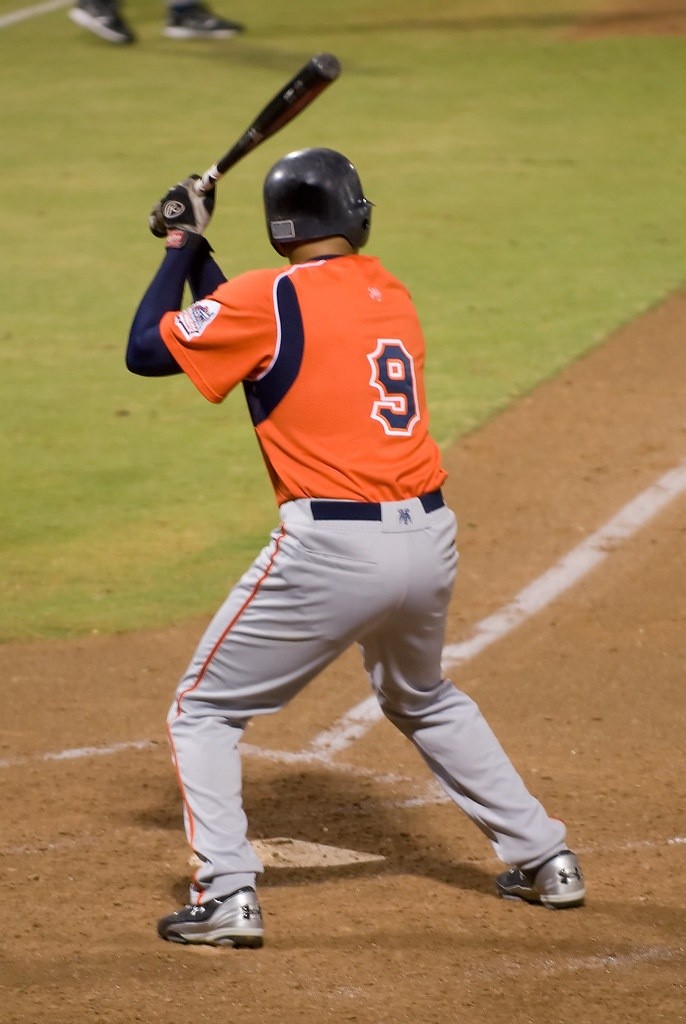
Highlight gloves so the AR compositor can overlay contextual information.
[160,174,217,251]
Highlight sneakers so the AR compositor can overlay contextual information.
[166,4,244,39]
[494,849,589,905]
[156,887,264,949]
[68,0,133,44]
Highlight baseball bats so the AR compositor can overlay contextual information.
[147,53,342,240]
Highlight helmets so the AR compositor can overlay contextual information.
[262,147,372,258]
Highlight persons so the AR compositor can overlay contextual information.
[68,0,246,46]
[124,147,591,947]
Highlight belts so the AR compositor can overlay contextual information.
[311,487,444,522]
